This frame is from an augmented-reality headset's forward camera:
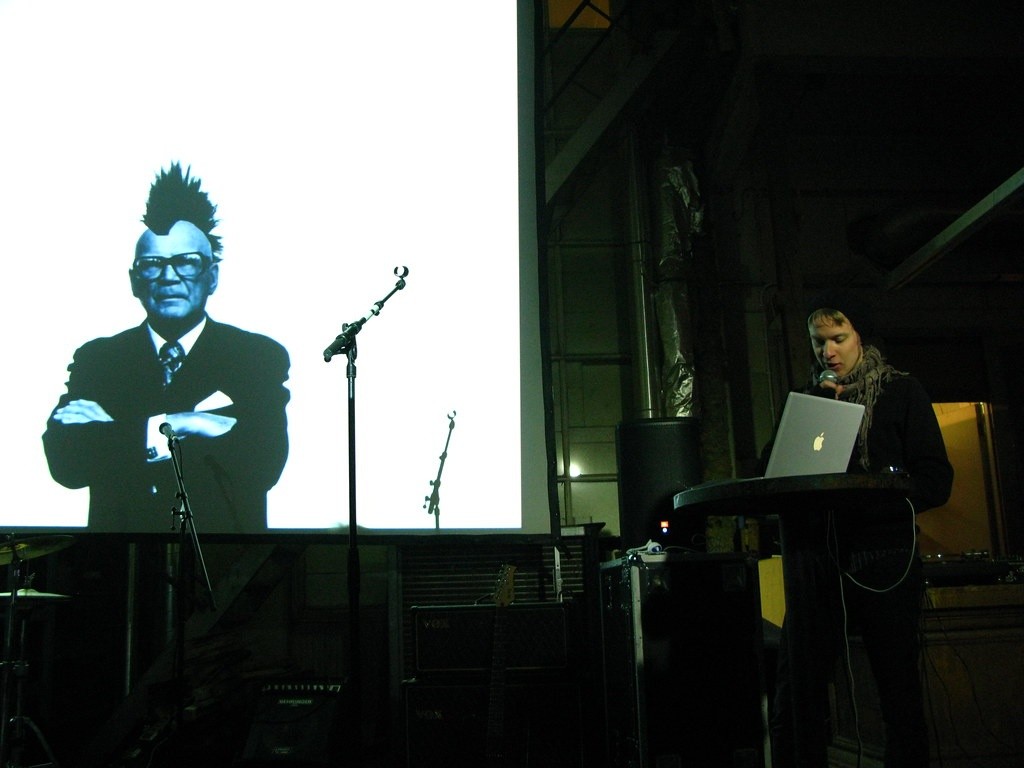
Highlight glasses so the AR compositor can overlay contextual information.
[133,251,214,281]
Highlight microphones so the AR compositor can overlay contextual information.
[819,370,837,398]
[158,422,179,443]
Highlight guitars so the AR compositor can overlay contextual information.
[453,563,543,768]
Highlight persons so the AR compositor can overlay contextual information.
[42,157,291,533]
[758,291,953,768]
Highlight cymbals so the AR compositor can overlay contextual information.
[0,588,71,598]
[0,534,79,565]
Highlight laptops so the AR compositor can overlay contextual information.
[692,391,865,488]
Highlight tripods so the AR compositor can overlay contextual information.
[0,601,58,768]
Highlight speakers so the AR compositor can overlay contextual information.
[403,677,590,768]
[615,416,705,555]
[233,683,366,768]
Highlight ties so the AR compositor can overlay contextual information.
[159,342,186,386]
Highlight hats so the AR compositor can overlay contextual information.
[806,290,871,345]
